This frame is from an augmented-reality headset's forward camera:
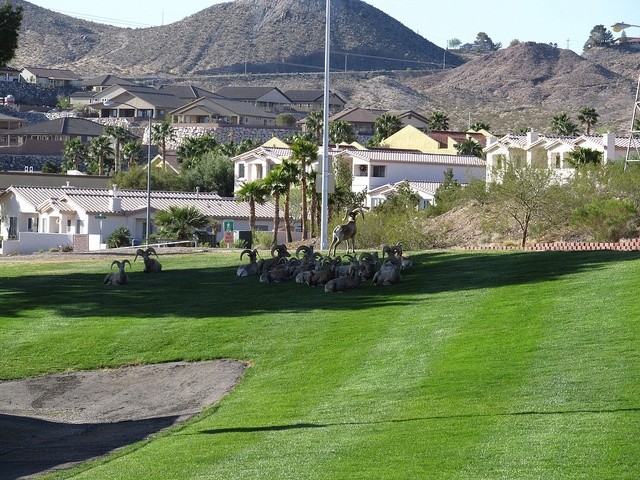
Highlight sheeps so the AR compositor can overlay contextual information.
[325,265,365,294]
[239,249,258,278]
[295,245,322,260]
[102,260,131,286]
[395,241,413,269]
[310,256,341,288]
[259,257,298,283]
[296,257,324,287]
[258,244,291,275]
[335,254,359,278]
[328,208,364,256]
[359,251,378,281]
[292,252,323,280]
[370,245,402,286]
[134,247,162,273]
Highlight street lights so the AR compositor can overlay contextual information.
[102,98,152,249]
